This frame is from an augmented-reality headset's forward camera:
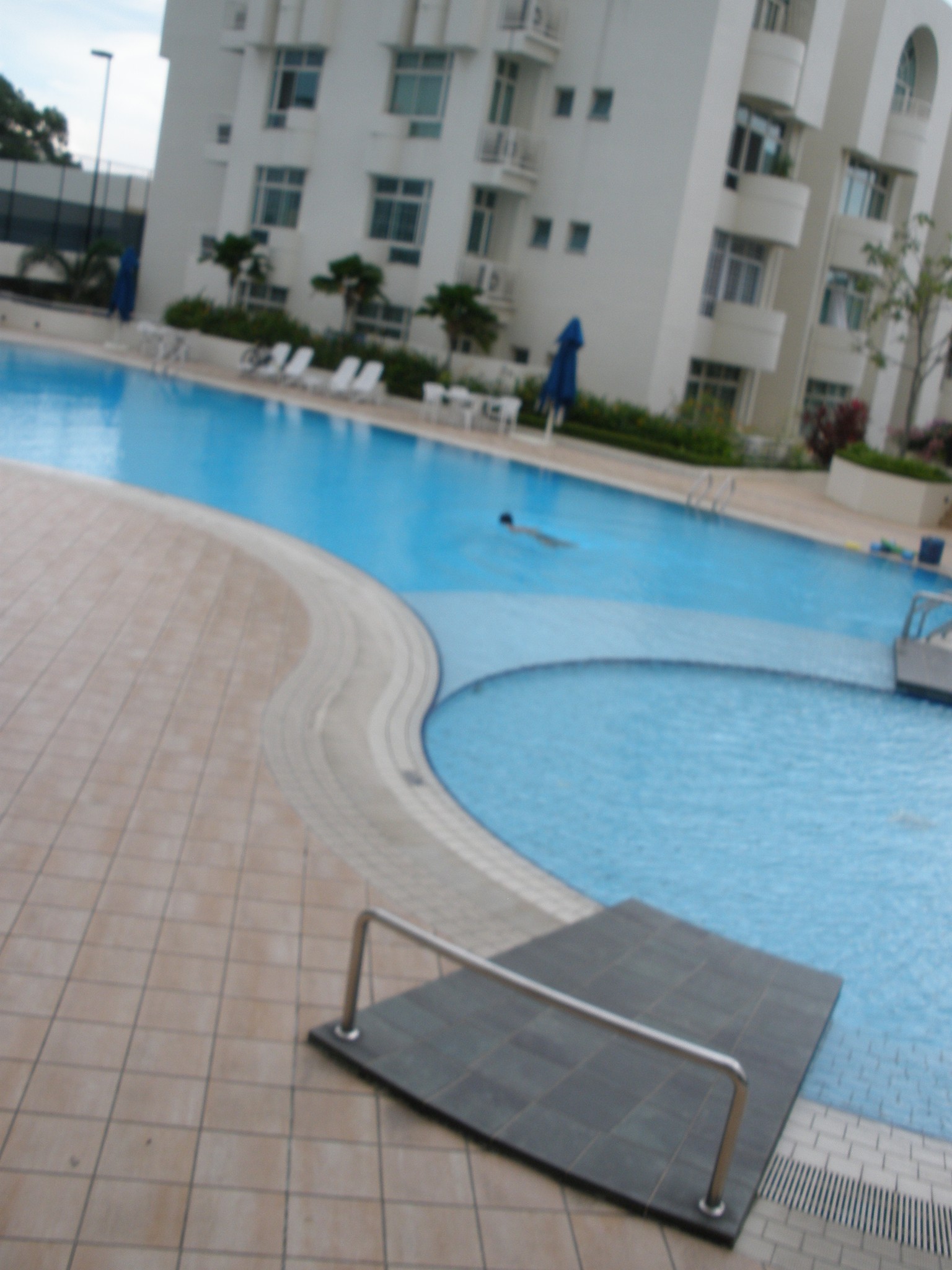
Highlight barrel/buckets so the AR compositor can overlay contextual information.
[918,536,944,564]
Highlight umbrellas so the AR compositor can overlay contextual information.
[103,245,140,349]
[534,318,584,442]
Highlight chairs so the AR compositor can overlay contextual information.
[258,343,520,439]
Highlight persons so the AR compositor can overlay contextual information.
[500,512,576,550]
